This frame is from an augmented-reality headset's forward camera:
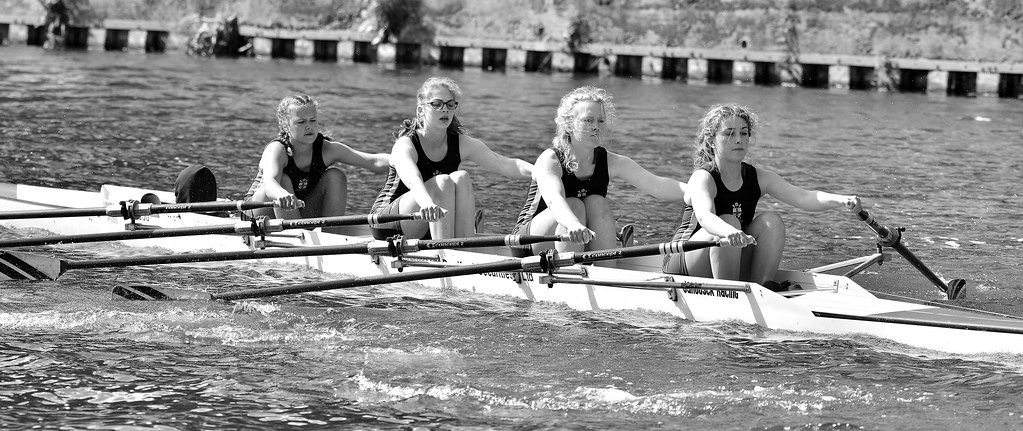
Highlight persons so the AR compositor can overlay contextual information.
[246,91,390,220]
[510,86,688,269]
[369,76,535,240]
[661,104,863,284]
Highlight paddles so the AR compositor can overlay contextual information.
[108,234,755,306]
[473,208,486,233]
[616,223,635,247]
[845,200,967,299]
[0,198,306,222]
[0,230,594,283]
[0,208,443,249]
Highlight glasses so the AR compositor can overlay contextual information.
[420,98,459,110]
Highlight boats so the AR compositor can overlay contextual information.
[0,182,1022,362]
